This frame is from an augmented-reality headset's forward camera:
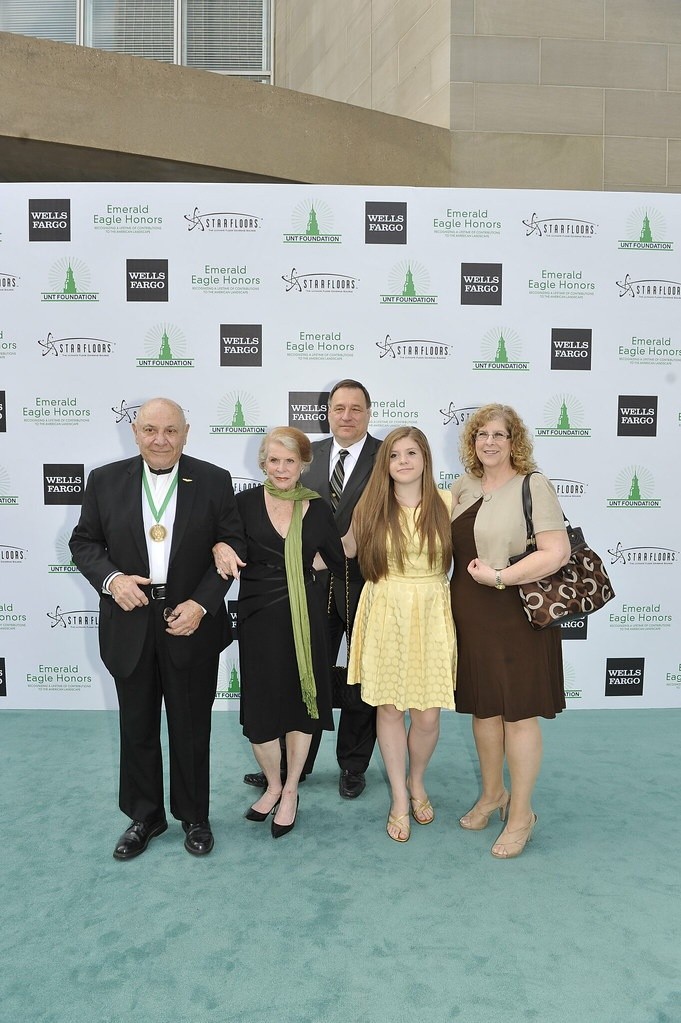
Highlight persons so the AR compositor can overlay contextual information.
[449,402,571,859]
[243,380,387,801]
[212,425,360,840]
[68,395,236,861]
[312,427,458,842]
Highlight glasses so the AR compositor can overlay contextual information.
[474,431,512,443]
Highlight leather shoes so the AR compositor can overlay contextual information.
[339,769,366,799]
[114,815,168,857]
[181,820,214,855]
[244,770,306,788]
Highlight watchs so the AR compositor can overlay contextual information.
[495,571,505,590]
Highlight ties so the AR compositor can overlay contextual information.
[329,449,350,514]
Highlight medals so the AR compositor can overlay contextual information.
[151,525,167,541]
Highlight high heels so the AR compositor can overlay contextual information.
[271,794,299,837]
[490,812,538,857]
[245,790,281,822]
[462,789,511,829]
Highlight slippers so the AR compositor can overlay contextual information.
[386,811,410,842]
[410,795,434,825]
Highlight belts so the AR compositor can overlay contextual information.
[150,585,166,599]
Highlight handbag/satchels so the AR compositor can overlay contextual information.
[511,472,616,629]
[328,666,360,708]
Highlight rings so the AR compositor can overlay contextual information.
[217,569,220,574]
[188,632,190,635]
